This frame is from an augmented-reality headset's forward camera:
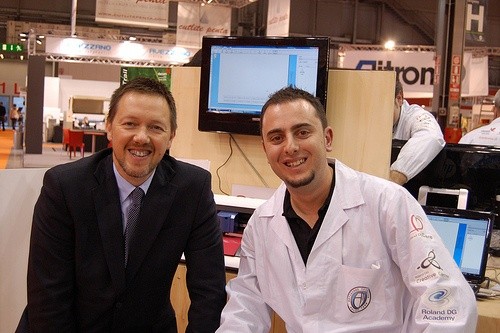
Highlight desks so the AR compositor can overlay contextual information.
[84,131,105,155]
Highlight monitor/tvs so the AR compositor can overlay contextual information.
[197,35,332,135]
[391,138,500,230]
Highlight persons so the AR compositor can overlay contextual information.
[15,77,228,333]
[389,79,446,186]
[457,88,500,148]
[9,103,19,131]
[0,101,6,131]
[214,89,477,333]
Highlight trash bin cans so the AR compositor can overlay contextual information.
[16,131,24,150]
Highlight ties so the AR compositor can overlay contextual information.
[123,188,146,273]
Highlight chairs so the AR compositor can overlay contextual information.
[63,128,85,160]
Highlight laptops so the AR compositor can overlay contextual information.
[419,204,495,295]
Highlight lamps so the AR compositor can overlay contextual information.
[37,34,44,39]
[20,37,28,42]
[36,38,42,45]
[18,32,28,37]
